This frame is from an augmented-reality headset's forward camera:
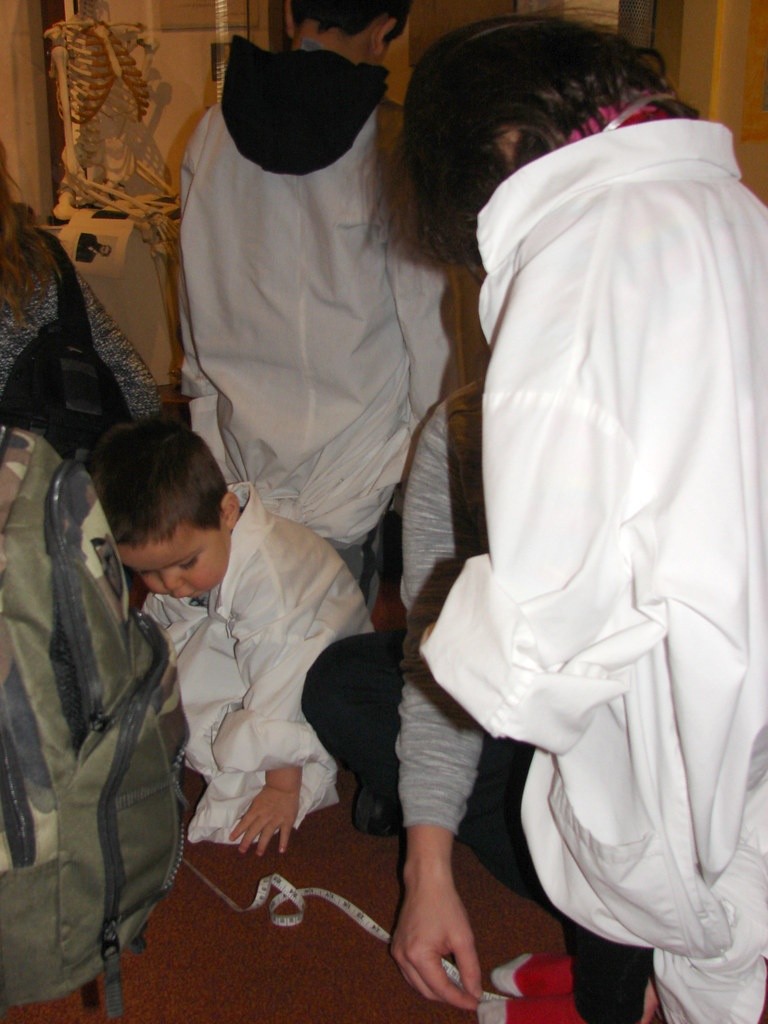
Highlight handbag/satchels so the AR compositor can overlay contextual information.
[1,228,133,461]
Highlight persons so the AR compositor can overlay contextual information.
[300,375,486,1011]
[177,1,459,614]
[403,19,768,1024]
[0,141,162,421]
[93,424,378,857]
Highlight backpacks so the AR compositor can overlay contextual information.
[0,421,191,1006]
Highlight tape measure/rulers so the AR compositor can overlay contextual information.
[178,855,508,1003]
